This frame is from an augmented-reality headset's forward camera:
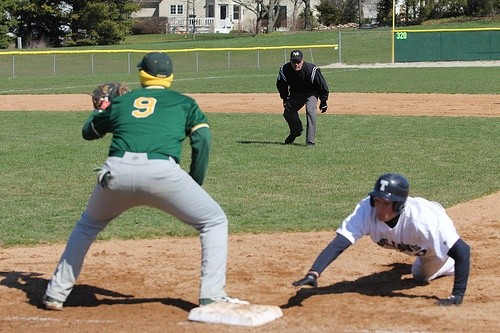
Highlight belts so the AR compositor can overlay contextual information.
[108,150,169,160]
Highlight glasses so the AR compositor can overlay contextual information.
[291,60,301,64]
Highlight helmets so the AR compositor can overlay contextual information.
[369,173,409,213]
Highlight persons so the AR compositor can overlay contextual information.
[41,52,250,312]
[276,50,328,146]
[292,174,470,307]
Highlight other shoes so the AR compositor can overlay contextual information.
[285,129,302,144]
[198,294,250,308]
[41,294,64,310]
[305,141,315,146]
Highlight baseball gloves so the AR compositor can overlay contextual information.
[92,82,131,109]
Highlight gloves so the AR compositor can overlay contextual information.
[282,99,292,113]
[291,273,318,289]
[433,296,463,306]
[320,103,327,113]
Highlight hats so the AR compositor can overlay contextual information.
[137,52,173,79]
[290,50,303,61]
